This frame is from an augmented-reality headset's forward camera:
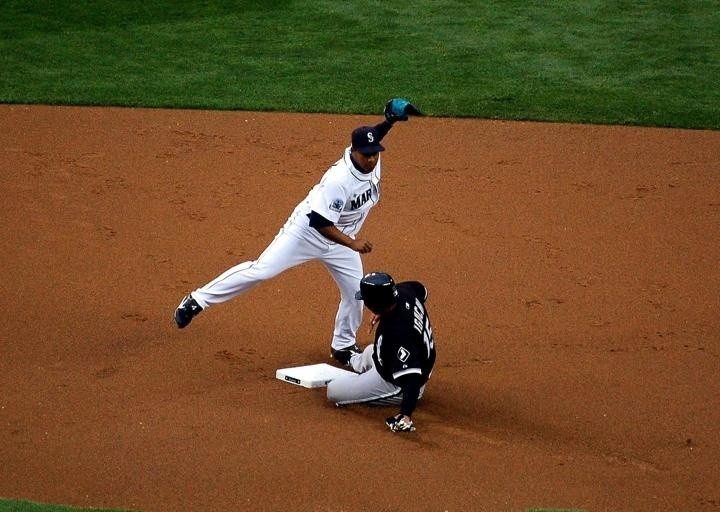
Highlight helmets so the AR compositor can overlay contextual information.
[354,272,400,310]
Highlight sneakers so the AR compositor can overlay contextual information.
[330,345,363,369]
[174,294,203,329]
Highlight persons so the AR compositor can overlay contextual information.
[324,268,439,433]
[174,96,426,365]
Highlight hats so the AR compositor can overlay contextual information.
[352,125,385,155]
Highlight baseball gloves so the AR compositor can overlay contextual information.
[384,98,427,120]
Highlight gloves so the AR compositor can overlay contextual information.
[386,415,416,433]
